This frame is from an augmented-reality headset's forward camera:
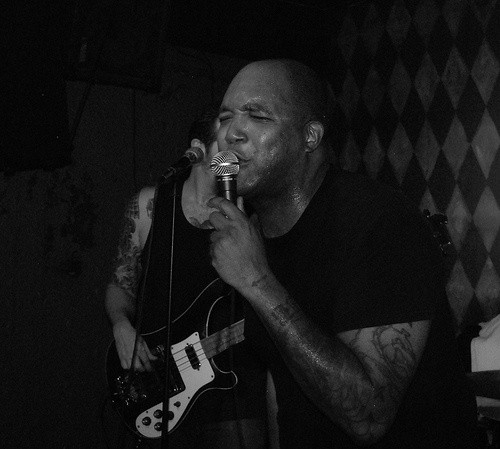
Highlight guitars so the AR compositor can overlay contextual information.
[105,277,244,442]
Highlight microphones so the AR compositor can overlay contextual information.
[159,147,203,182]
[210,151,240,219]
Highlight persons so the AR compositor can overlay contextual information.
[203,60,489,449]
[102,105,281,448]
